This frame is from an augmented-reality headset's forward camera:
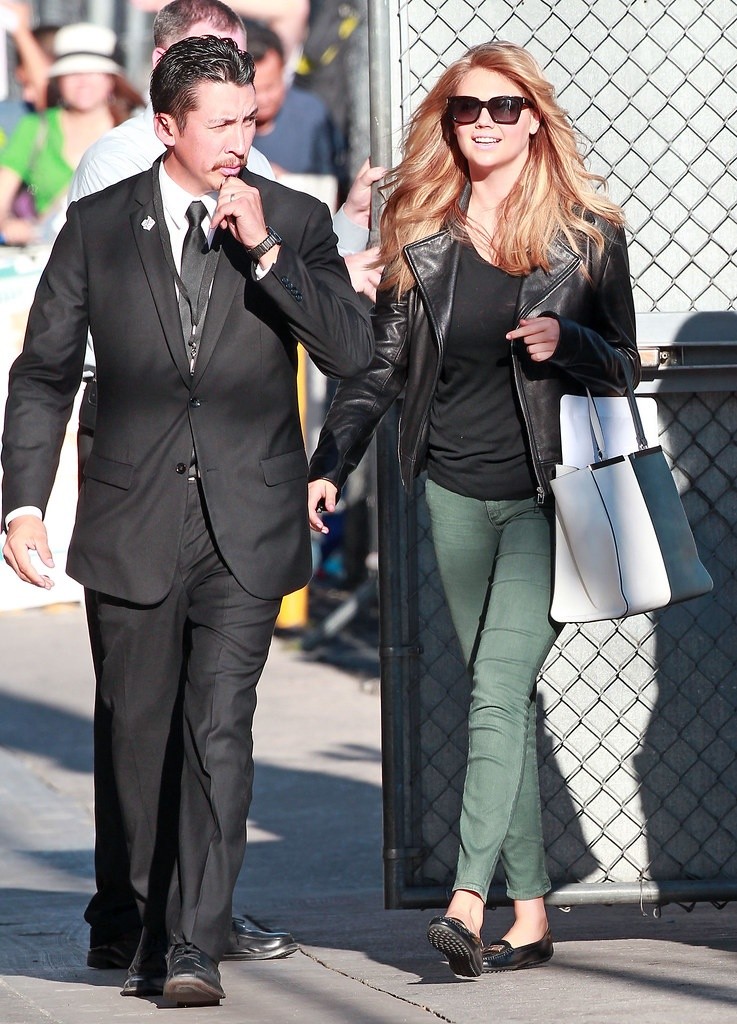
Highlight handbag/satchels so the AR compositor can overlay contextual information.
[548,349,714,624]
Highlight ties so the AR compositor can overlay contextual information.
[177,201,213,478]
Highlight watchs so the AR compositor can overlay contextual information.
[248,224,282,260]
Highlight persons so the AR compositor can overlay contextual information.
[0,37,373,1008]
[304,43,643,978]
[0,0,381,962]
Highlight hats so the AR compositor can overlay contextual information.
[46,23,122,77]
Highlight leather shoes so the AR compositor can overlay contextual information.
[121,973,167,996]
[162,941,227,1007]
[482,927,554,973]
[221,917,299,961]
[87,939,138,968]
[426,916,486,977]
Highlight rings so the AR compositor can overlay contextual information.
[230,194,235,202]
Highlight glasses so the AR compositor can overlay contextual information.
[446,95,534,124]
